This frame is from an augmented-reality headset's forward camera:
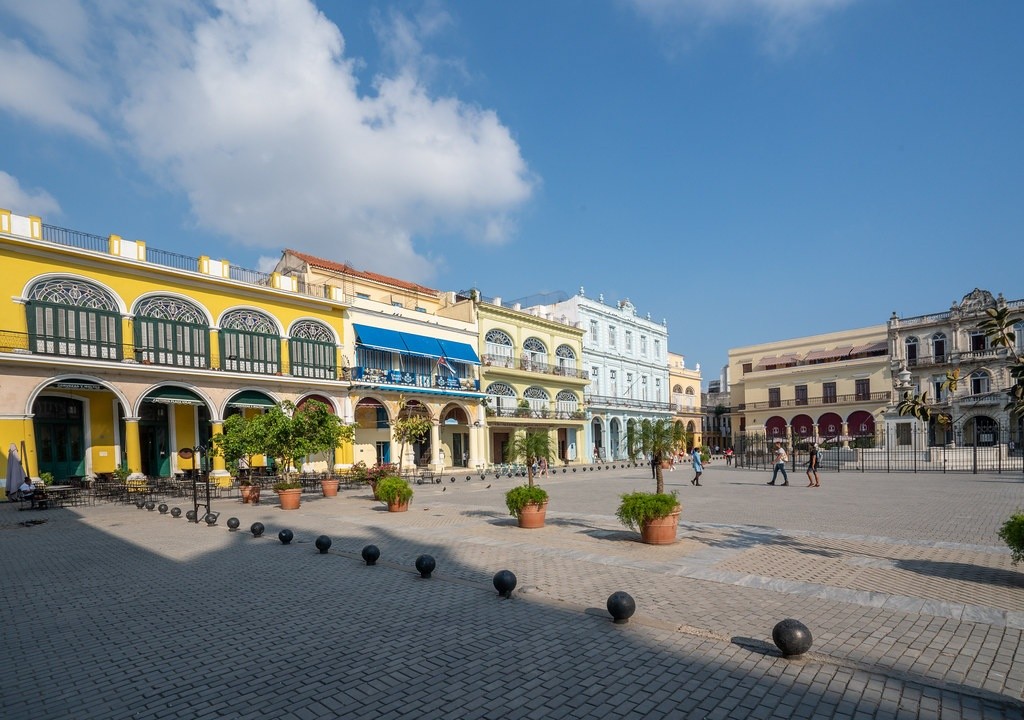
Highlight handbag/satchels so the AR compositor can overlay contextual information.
[777,448,788,463]
[693,462,695,468]
[538,458,542,466]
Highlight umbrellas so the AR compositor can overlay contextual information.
[6,443,27,502]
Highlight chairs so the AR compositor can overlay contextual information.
[476,461,555,476]
[15,466,444,510]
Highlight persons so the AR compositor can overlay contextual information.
[648,452,656,479]
[723,447,733,467]
[678,448,684,464]
[530,455,538,477]
[668,451,676,472]
[19,476,36,509]
[462,450,468,468]
[802,441,823,487]
[690,447,705,486]
[538,454,550,480]
[767,442,789,487]
[238,455,253,485]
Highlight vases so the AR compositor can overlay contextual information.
[371,484,382,501]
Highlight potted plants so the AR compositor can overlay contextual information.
[485,406,496,417]
[515,398,532,418]
[370,391,440,512]
[504,427,559,528]
[203,413,267,505]
[243,399,330,510]
[661,452,670,469]
[313,404,363,496]
[700,445,711,465]
[572,403,587,420]
[614,418,693,545]
[541,405,547,418]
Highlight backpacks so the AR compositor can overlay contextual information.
[813,450,823,462]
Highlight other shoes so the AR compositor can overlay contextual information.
[807,482,814,487]
[691,480,695,485]
[696,483,702,486]
[767,480,775,485]
[781,481,789,486]
[813,484,820,487]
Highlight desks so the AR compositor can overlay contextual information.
[51,487,81,509]
[199,484,220,500]
[298,478,322,493]
[94,483,121,500]
[115,485,135,504]
[419,471,436,484]
[45,485,74,505]
[134,485,158,502]
[258,476,279,490]
[177,480,193,496]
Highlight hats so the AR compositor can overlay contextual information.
[810,442,815,446]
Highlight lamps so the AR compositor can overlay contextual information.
[135,347,144,353]
[343,367,350,372]
[474,421,479,426]
[328,366,336,372]
[229,355,238,360]
[157,408,164,418]
[67,388,77,416]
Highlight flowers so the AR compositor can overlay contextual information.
[365,462,399,486]
